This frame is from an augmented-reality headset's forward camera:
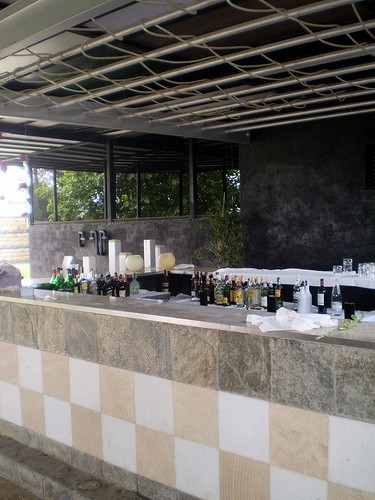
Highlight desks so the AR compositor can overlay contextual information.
[213,267,375,320]
[126,263,196,295]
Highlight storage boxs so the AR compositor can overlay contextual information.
[62,237,170,276]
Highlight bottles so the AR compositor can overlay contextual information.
[330,279,342,316]
[189,268,276,312]
[276,278,283,312]
[161,270,169,293]
[305,281,311,313]
[293,274,301,311]
[317,278,328,314]
[298,282,306,313]
[48,267,138,297]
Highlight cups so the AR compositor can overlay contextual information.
[333,266,342,278]
[342,258,352,271]
[357,262,375,279]
